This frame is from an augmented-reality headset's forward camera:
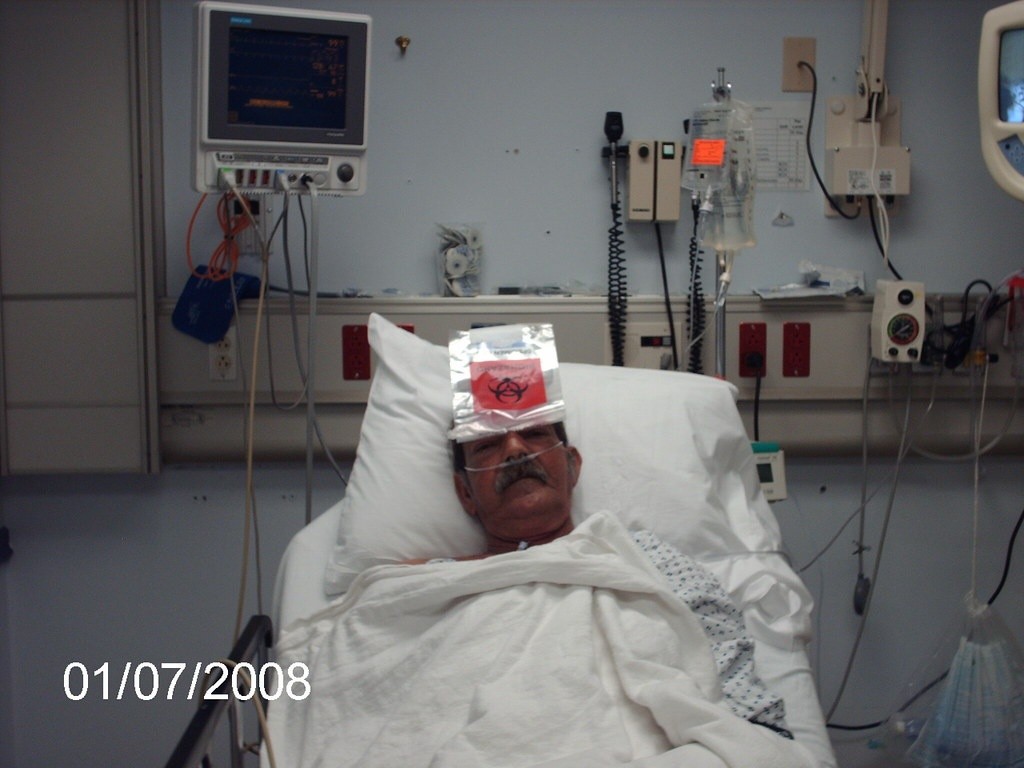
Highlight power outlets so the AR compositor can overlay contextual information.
[783,322,811,377]
[209,326,237,382]
[343,324,371,380]
[739,323,766,377]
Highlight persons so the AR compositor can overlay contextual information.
[398,400,795,740]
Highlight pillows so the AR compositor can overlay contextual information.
[324,312,741,599]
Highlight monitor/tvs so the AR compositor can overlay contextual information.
[195,2,373,155]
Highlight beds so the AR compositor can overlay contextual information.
[166,497,840,768]
